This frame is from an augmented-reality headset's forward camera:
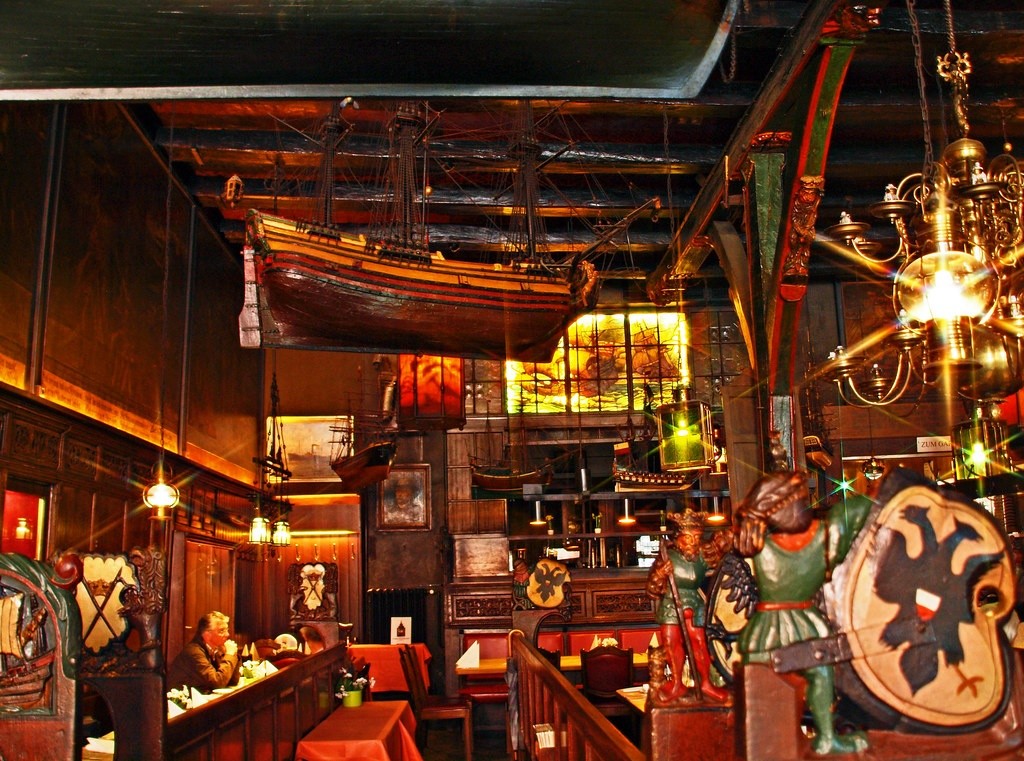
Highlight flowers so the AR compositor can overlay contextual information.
[334,668,376,698]
[592,511,603,528]
[545,515,554,530]
[660,510,666,526]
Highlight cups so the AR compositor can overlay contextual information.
[238,677,246,683]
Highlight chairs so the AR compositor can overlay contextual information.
[397,644,474,761]
[579,644,638,743]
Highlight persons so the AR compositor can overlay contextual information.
[253,628,324,663]
[171,611,241,691]
[646,472,872,754]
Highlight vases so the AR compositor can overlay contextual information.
[547,530,554,535]
[343,689,362,707]
[594,528,602,534]
[660,526,667,531]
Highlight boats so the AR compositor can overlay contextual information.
[467,454,554,494]
[244,205,601,364]
[611,463,706,495]
[325,416,400,495]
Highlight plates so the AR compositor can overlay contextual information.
[212,688,233,693]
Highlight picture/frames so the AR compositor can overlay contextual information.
[375,463,432,532]
[265,414,354,483]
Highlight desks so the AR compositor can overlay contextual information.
[455,651,649,677]
[297,701,423,761]
[616,687,647,712]
[351,644,431,694]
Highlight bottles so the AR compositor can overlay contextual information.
[397,620,405,636]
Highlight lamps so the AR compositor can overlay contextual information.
[818,0,1024,407]
[141,459,180,522]
[707,496,724,521]
[654,374,715,472]
[245,346,293,547]
[528,501,546,525]
[949,416,1014,482]
[617,499,636,523]
